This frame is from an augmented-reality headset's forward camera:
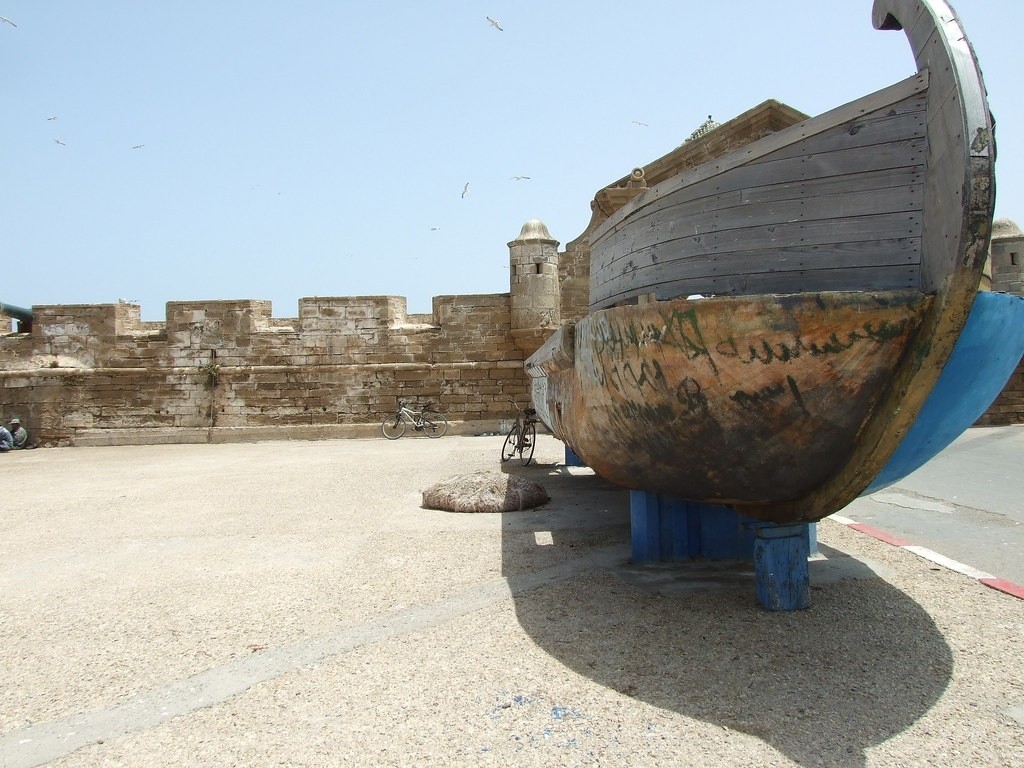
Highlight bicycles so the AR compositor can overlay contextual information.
[382,401,448,440]
[502,399,537,467]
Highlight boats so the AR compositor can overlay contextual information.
[511,0,997,530]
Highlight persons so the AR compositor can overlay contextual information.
[8,419,28,450]
[0,425,13,452]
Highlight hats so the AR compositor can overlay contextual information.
[8,419,20,424]
